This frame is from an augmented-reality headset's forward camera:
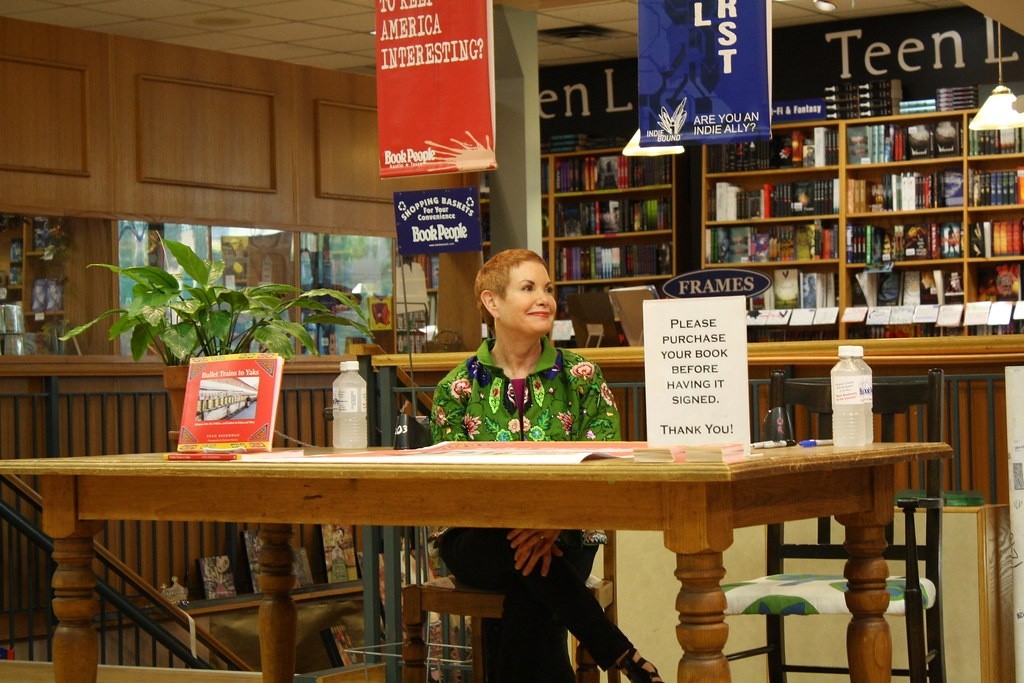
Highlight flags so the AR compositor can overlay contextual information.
[639,0,773,148]
[375,0,499,181]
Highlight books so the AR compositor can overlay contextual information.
[164,448,304,461]
[177,351,286,454]
[633,444,747,465]
[966,117,1024,336]
[704,126,838,343]
[844,115,965,340]
[535,153,673,323]
[203,525,357,666]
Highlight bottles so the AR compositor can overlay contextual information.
[332,362,346,449]
[831,346,866,446]
[338,361,368,449]
[851,346,873,444]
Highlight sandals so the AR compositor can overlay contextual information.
[616,648,664,683]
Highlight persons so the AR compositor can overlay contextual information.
[431,247,662,683]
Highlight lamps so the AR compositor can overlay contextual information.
[968,22,1024,130]
[622,120,685,156]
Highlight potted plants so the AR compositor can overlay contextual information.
[57,236,374,442]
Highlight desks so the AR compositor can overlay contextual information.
[0,443,954,683]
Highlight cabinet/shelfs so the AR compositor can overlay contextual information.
[0,212,63,355]
[541,110,1024,343]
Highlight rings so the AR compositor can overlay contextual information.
[540,532,544,538]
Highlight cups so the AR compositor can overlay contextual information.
[0,304,38,355]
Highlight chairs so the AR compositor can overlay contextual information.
[721,368,947,683]
[400,531,621,683]
